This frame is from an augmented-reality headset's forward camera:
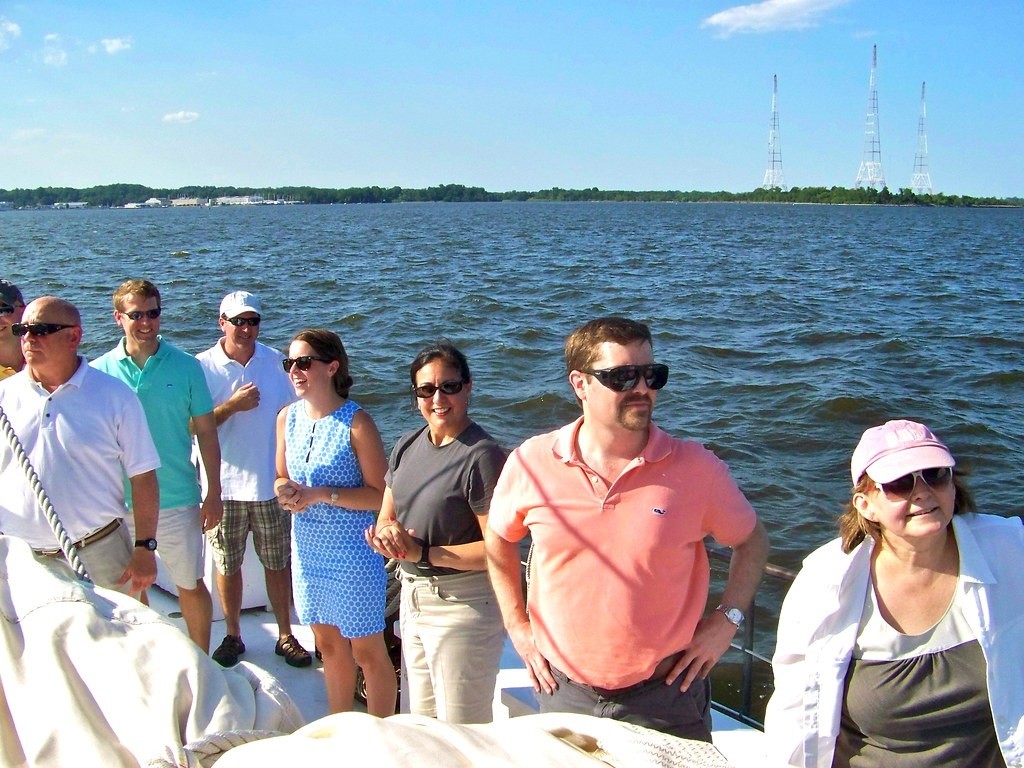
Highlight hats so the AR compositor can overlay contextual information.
[0,279,25,306]
[851,420,955,488]
[219,291,262,320]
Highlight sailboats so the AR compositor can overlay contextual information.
[228,192,306,206]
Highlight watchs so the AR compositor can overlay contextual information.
[330,486,339,507]
[716,604,746,630]
[135,538,158,551]
[415,545,432,569]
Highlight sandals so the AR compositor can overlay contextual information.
[275,634,313,667]
[212,634,245,667]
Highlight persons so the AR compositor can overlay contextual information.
[0,277,26,381]
[0,295,162,602]
[365,335,507,724]
[766,419,1024,768]
[485,316,771,744]
[89,279,224,657]
[274,329,397,719]
[190,290,313,667]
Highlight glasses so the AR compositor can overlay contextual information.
[0,305,24,316]
[11,323,77,337]
[872,466,953,502]
[412,378,466,398]
[222,316,261,326]
[282,355,325,373]
[584,364,668,392]
[121,305,161,320]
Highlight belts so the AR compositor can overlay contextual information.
[34,517,124,558]
